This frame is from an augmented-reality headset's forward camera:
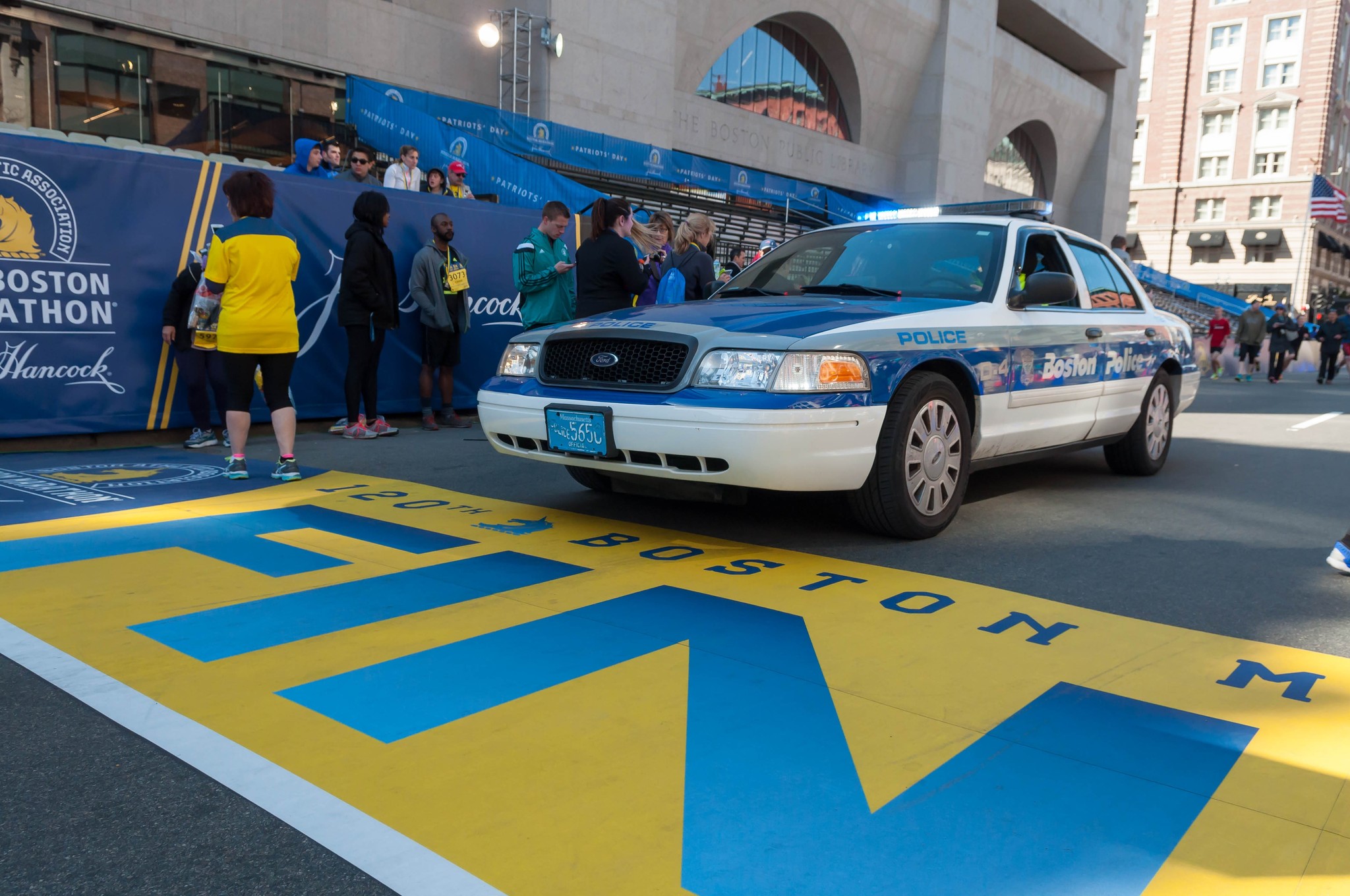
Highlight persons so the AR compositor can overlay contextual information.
[1111,234,1133,272]
[631,210,674,307]
[203,170,301,481]
[1233,299,1350,385]
[762,257,796,291]
[338,191,399,439]
[956,242,1026,292]
[282,138,475,201]
[749,239,777,265]
[410,213,472,430]
[512,201,576,332]
[1326,526,1350,572]
[1204,307,1231,380]
[661,212,731,303]
[725,249,745,278]
[162,262,231,449]
[575,197,667,319]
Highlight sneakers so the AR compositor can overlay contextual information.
[440,411,473,428]
[184,427,218,448]
[1209,374,1219,380]
[222,429,230,447]
[364,419,399,436]
[422,412,439,430]
[342,414,378,439]
[222,454,249,479]
[271,454,302,481]
[1216,367,1225,377]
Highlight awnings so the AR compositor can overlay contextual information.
[1186,230,1225,247]
[1118,233,1137,248]
[1241,229,1281,245]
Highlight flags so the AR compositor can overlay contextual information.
[1309,175,1348,223]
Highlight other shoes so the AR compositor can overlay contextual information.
[1326,543,1349,572]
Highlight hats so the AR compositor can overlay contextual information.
[759,239,777,250]
[448,161,466,174]
[1275,302,1286,310]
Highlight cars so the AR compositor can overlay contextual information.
[475,197,1202,543]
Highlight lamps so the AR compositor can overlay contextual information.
[541,19,563,57]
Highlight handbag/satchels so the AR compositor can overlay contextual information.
[1283,318,1299,342]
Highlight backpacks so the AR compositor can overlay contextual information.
[655,249,699,305]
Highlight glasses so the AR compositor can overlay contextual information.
[456,173,466,178]
[650,227,668,234]
[351,157,369,165]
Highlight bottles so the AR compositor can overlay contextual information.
[463,189,469,198]
[713,259,721,280]
[638,259,645,272]
